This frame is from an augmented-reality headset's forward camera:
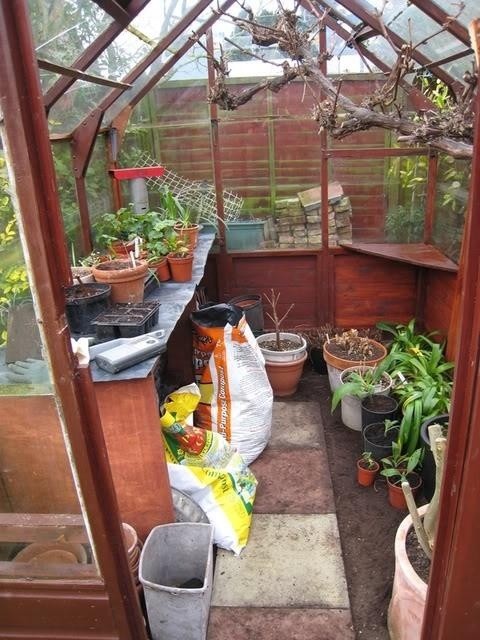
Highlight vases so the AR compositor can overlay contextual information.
[255,332,308,399]
[385,504,444,640]
[11,520,144,603]
[228,293,265,339]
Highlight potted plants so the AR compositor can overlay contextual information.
[61,183,201,342]
[312,316,455,510]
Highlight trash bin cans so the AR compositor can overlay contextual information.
[138,522,214,640]
